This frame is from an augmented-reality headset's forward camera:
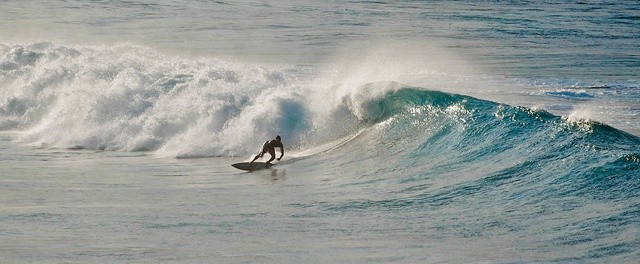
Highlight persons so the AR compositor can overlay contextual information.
[248,135,285,167]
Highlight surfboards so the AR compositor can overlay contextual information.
[233,163,273,169]
